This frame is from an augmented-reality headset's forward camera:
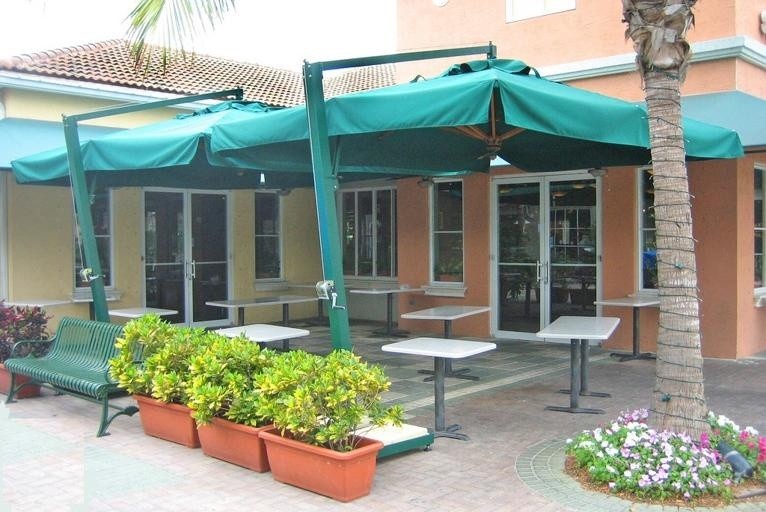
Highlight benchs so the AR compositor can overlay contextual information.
[4,315,139,437]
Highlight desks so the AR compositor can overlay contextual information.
[381,336,495,441]
[109,307,180,324]
[205,296,319,353]
[350,288,423,338]
[536,313,620,415]
[593,295,660,362]
[156,279,227,319]
[213,321,310,353]
[400,307,492,336]
[290,282,332,324]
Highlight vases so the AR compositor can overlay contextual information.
[438,274,462,281]
[0,364,41,398]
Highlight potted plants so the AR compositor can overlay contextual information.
[259,349,405,504]
[108,313,200,447]
[499,222,535,304]
[534,256,570,302]
[569,257,596,304]
[185,333,275,473]
[647,270,657,288]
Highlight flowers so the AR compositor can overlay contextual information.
[437,249,464,273]
[0,302,52,362]
